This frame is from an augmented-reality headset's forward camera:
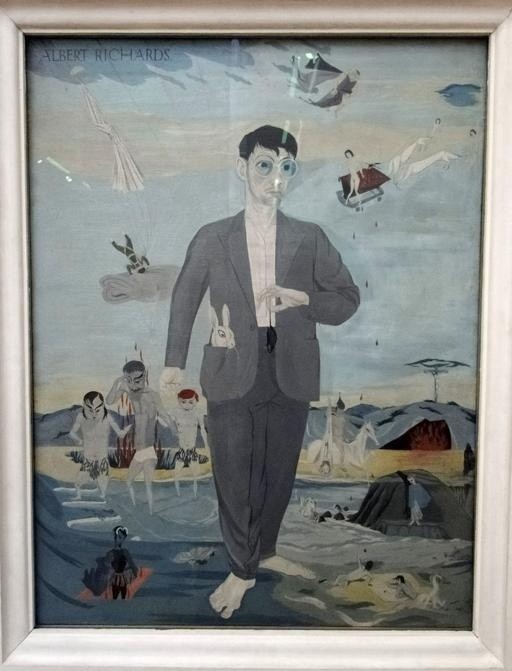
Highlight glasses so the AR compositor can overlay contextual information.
[247,157,298,180]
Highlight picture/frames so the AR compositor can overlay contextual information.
[0,0,512,671]
